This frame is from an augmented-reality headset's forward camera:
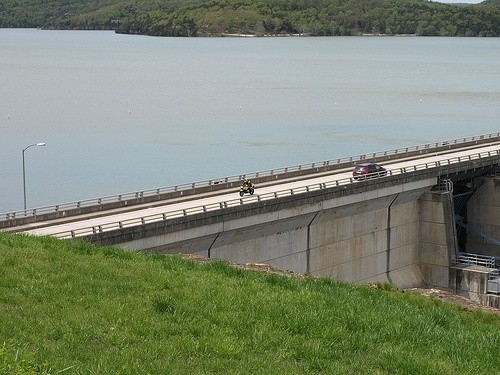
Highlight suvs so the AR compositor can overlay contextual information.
[353,163,387,180]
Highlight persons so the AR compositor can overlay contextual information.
[242,178,251,192]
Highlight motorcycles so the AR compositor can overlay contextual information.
[239,182,255,197]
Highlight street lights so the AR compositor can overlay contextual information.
[22,142,46,217]
